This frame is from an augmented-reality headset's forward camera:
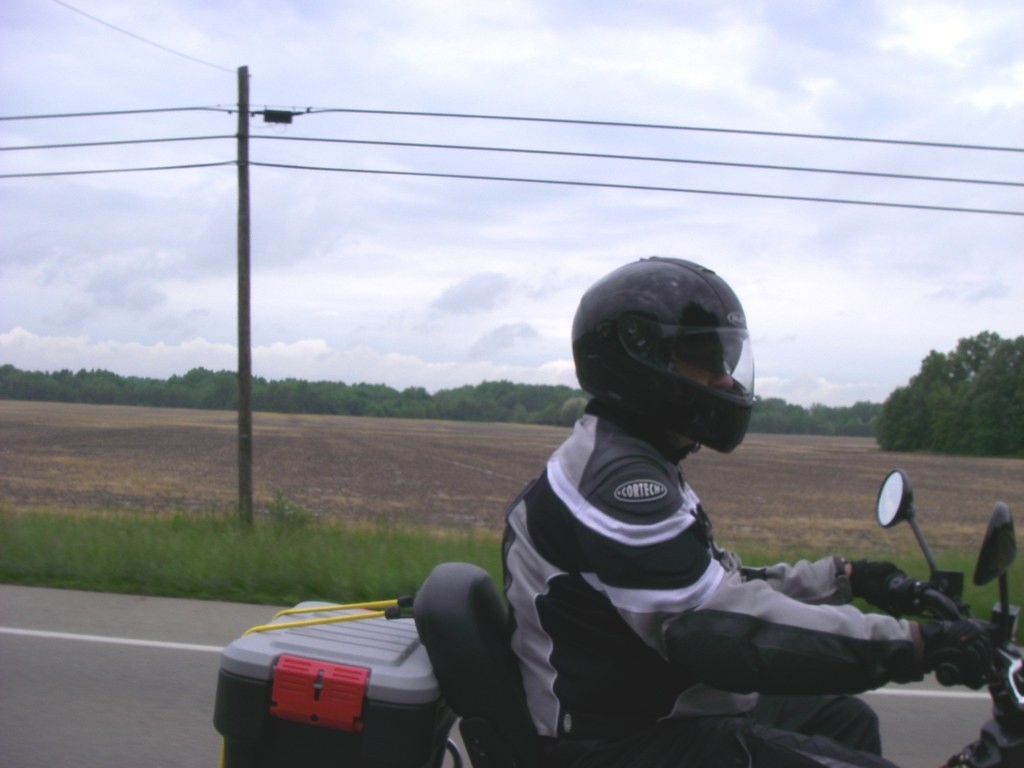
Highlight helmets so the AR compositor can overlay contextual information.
[572,258,756,455]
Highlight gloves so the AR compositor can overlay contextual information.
[918,619,996,689]
[847,561,916,619]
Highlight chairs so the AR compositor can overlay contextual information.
[418,563,542,768]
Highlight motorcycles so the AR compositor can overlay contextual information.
[213,465,1023,768]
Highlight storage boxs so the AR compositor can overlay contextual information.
[212,600,460,767]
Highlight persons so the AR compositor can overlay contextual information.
[501,250,995,768]
